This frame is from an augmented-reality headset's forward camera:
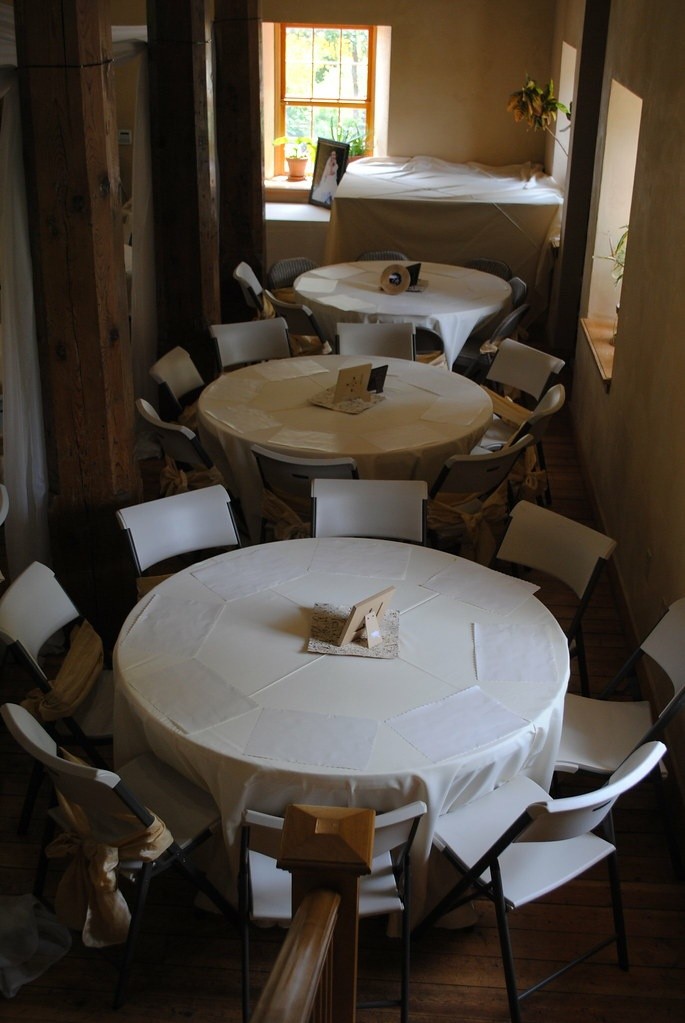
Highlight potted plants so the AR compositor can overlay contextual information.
[330,116,372,166]
[271,135,316,181]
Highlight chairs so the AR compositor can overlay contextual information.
[0,248,685,1023]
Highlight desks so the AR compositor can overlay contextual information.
[113,537,571,940]
[295,259,512,370]
[197,354,494,550]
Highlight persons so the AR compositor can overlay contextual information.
[312,150,338,205]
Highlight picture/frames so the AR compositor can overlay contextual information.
[308,135,350,209]
[337,587,396,647]
